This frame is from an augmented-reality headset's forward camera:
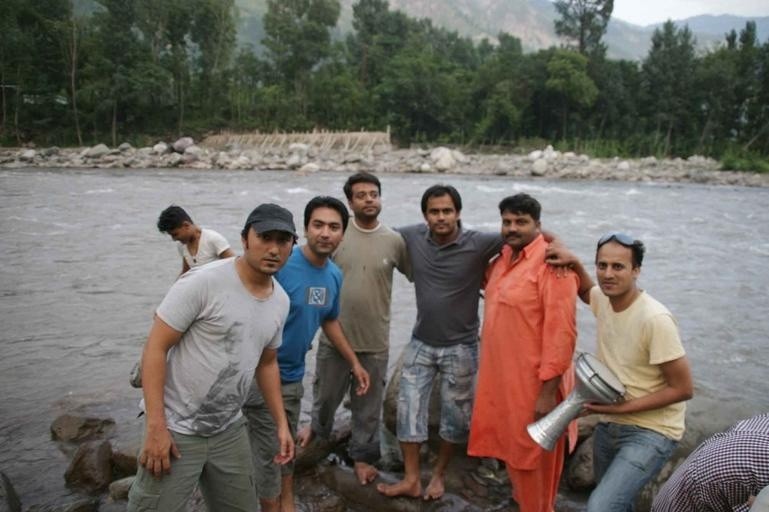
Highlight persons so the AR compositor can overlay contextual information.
[463,190,580,512]
[375,183,579,502]
[238,195,372,511]
[650,413,769,511]
[541,231,696,512]
[123,203,298,512]
[296,169,406,486]
[154,204,235,278]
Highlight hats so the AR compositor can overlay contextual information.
[243,203,300,240]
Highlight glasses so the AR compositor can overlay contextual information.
[596,231,642,252]
